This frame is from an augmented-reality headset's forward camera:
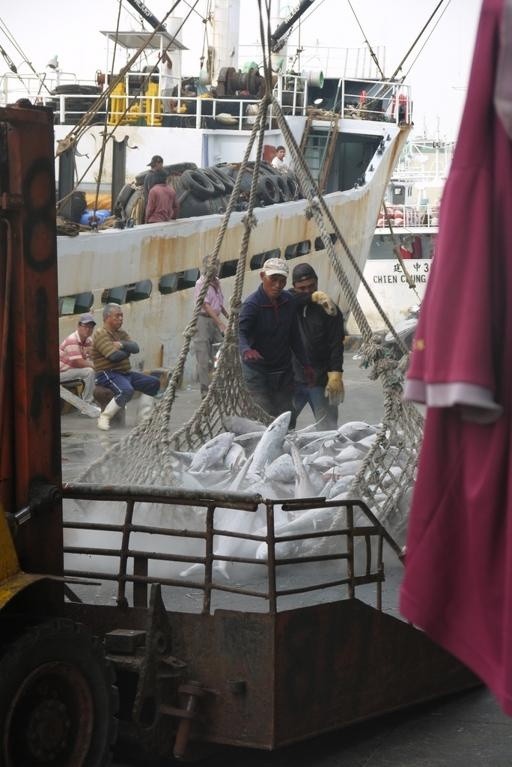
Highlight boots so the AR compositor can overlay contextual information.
[97,397,122,431]
[135,393,155,426]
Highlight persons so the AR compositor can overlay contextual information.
[142,154,165,215]
[271,144,289,171]
[89,302,160,430]
[237,258,317,428]
[286,263,345,431]
[142,170,180,223]
[194,256,229,400]
[60,312,97,415]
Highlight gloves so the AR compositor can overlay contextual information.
[243,348,265,362]
[323,371,345,407]
[303,363,318,389]
[311,290,337,316]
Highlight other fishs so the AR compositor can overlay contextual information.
[158,408,419,581]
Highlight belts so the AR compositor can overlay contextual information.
[198,312,212,318]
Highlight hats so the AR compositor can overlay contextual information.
[78,313,96,326]
[263,258,289,280]
[146,156,163,166]
